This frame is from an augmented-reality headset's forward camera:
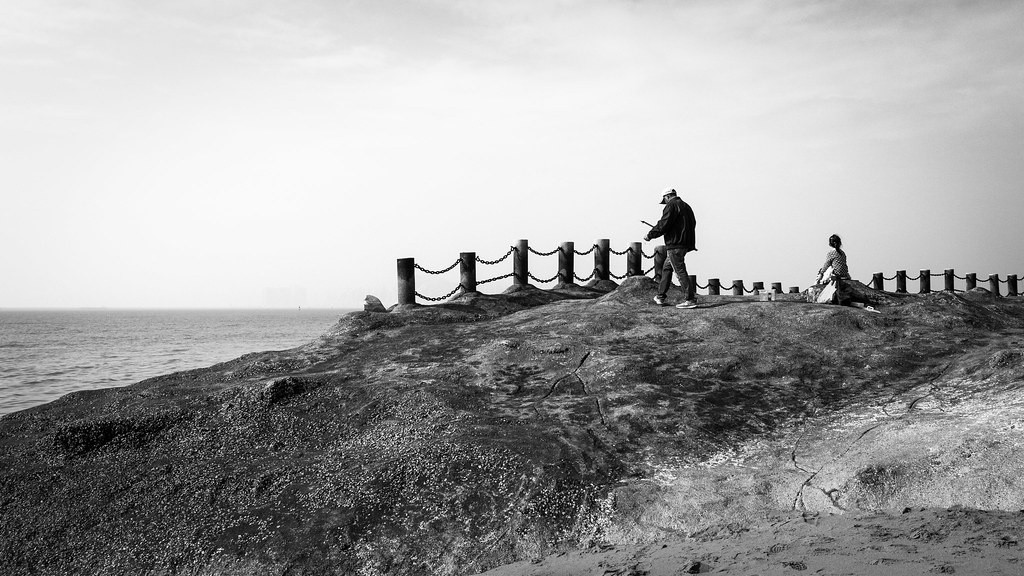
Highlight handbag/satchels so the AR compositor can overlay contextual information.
[816,280,837,303]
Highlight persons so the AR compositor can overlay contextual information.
[645,188,699,310]
[817,235,851,284]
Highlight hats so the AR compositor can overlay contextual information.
[659,188,676,204]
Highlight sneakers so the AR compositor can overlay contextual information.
[676,300,697,309]
[654,295,667,304]
